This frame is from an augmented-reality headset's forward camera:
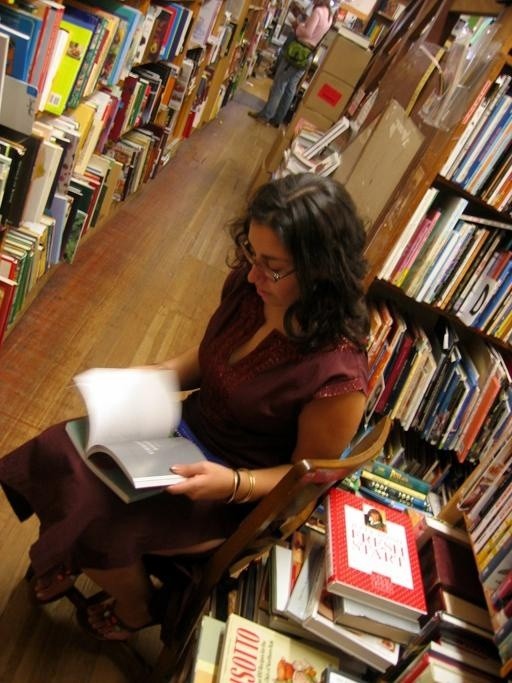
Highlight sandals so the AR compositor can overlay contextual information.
[26,563,84,602]
[76,588,163,641]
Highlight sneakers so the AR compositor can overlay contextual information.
[248,110,279,128]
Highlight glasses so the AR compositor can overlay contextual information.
[236,234,297,283]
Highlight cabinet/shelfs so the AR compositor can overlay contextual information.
[1,0,510,681]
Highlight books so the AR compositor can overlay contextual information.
[366,4,407,45]
[65,411,211,506]
[379,74,512,345]
[193,302,512,683]
[74,363,211,490]
[0,0,274,333]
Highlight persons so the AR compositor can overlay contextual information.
[2,171,371,644]
[249,0,335,131]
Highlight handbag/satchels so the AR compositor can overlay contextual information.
[285,41,312,69]
